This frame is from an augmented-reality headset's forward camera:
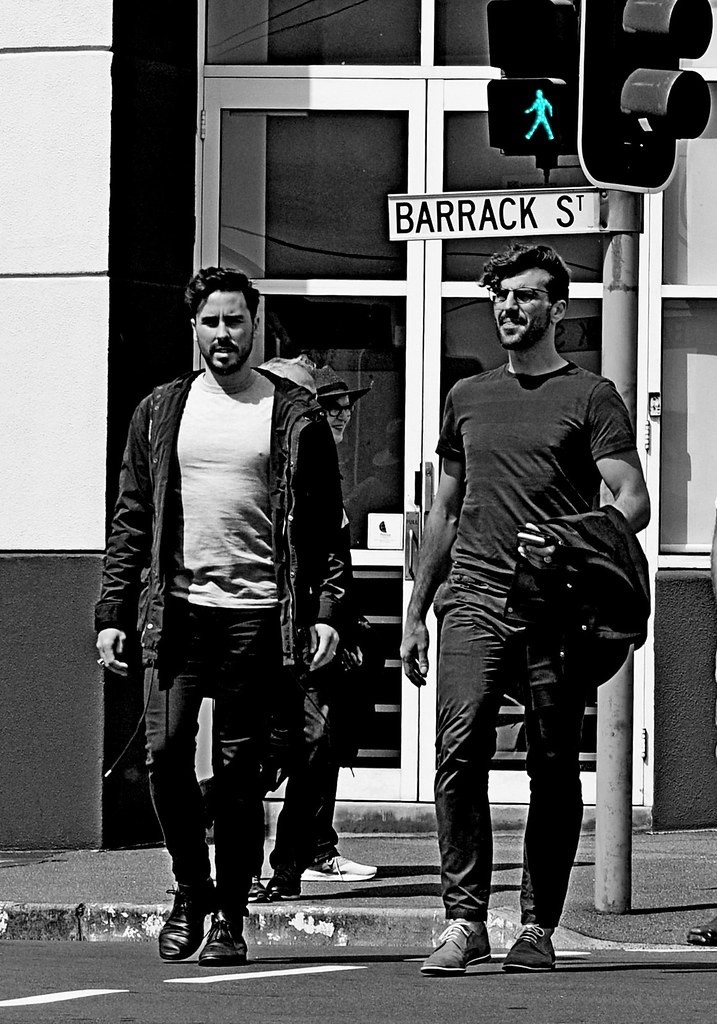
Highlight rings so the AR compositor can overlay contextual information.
[97,658,105,667]
[332,651,337,656]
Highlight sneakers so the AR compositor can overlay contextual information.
[246,876,267,902]
[300,855,377,881]
[268,859,301,900]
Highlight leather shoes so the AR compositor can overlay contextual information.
[502,922,556,972]
[198,908,247,965]
[421,918,489,973]
[157,876,215,961]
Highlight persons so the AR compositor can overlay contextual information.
[188,357,374,879]
[89,261,355,966]
[300,366,380,882]
[390,245,657,975]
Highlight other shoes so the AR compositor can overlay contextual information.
[687,914,716,946]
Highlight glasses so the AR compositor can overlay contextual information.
[488,286,550,304]
[320,402,355,417]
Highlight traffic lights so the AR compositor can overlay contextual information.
[481,0,579,150]
[580,0,713,194]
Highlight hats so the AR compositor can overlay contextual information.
[308,364,372,402]
